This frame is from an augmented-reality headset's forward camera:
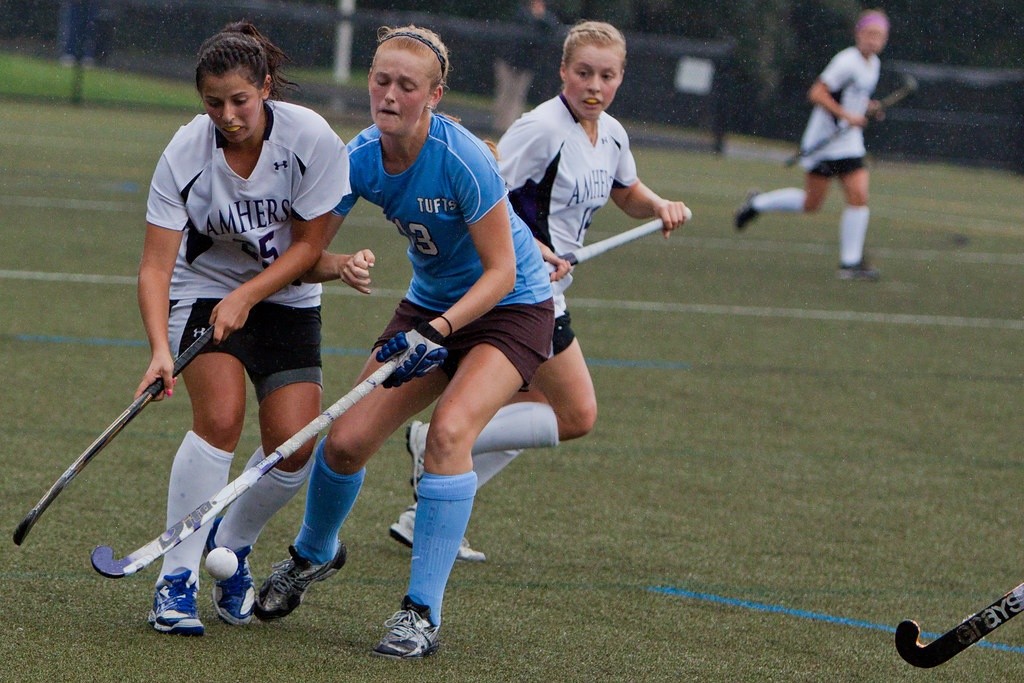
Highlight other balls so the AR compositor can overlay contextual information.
[206,546,238,579]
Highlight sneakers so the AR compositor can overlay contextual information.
[734,187,761,234]
[389,503,487,562]
[253,538,348,622]
[147,569,205,637]
[370,596,440,661]
[836,256,880,282]
[407,420,432,502]
[202,515,256,626]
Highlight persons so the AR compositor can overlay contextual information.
[387,20,685,561]
[487,1,560,143]
[252,20,559,661]
[135,23,356,639]
[729,10,895,284]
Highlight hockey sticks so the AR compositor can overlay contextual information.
[893,579,1024,668]
[544,204,690,276]
[90,347,410,577]
[782,74,918,168]
[11,324,215,547]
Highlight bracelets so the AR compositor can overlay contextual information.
[438,315,453,337]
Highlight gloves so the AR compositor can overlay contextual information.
[374,321,448,389]
